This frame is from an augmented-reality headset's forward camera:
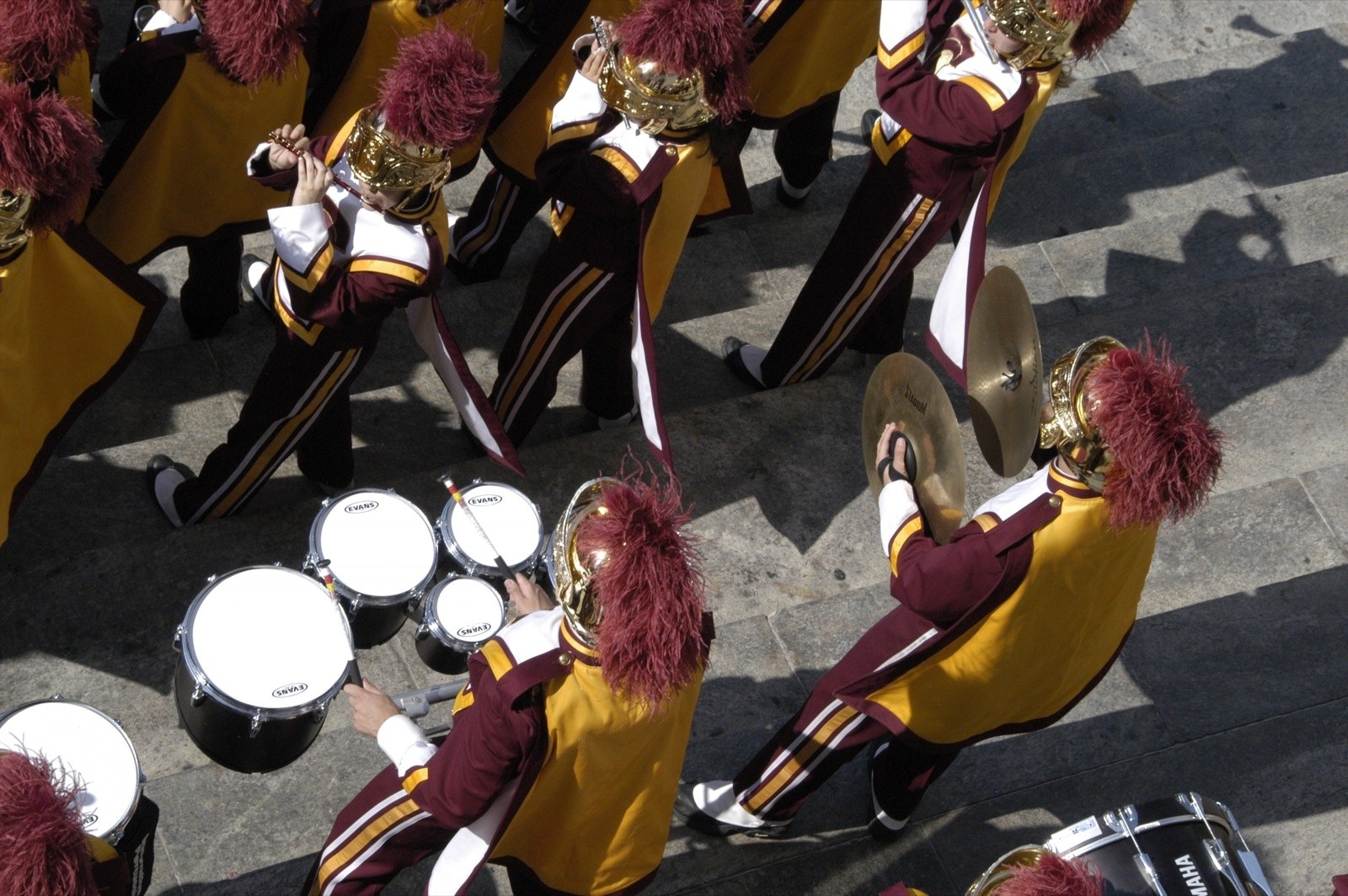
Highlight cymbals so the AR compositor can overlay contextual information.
[965,264,1045,480]
[861,352,965,545]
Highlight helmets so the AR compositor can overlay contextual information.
[1,86,97,255]
[1038,333,1222,531]
[983,0,1124,71]
[344,25,501,215]
[598,0,750,139]
[549,475,703,704]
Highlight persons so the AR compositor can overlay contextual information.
[876,844,1106,896]
[672,327,1231,841]
[0,734,132,896]
[298,444,714,896]
[0,0,1134,529]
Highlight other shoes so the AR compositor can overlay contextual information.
[147,452,189,528]
[672,781,793,839]
[241,253,283,321]
[722,334,769,390]
[776,166,813,209]
[866,735,911,845]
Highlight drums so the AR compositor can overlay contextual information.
[170,564,355,777]
[1039,790,1275,895]
[539,531,562,602]
[414,575,506,677]
[433,480,547,605]
[306,486,440,653]
[0,695,147,846]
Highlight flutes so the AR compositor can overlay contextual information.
[268,130,387,216]
[963,0,999,65]
[591,14,632,132]
